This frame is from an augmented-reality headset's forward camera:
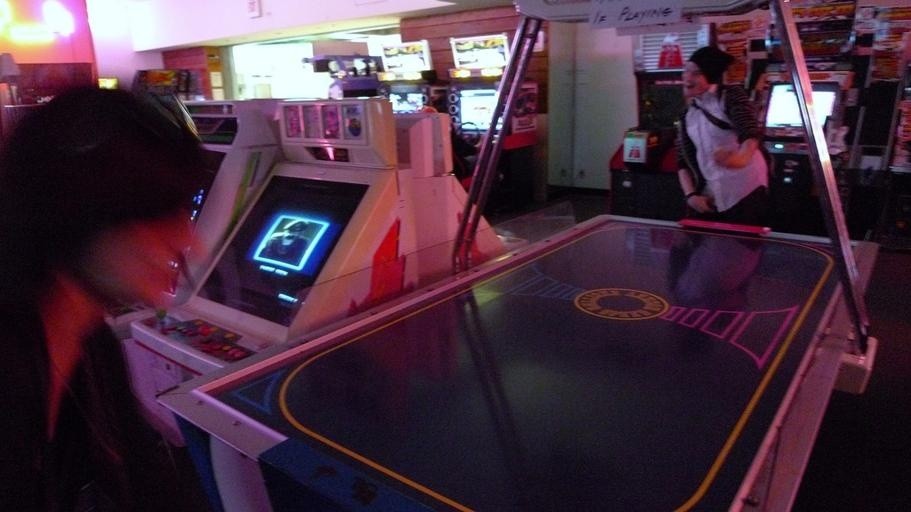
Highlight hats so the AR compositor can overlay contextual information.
[686,46,734,84]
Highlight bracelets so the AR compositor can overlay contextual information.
[684,192,698,200]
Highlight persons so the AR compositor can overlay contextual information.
[0,87,215,512]
[674,47,769,228]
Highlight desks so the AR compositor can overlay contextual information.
[153,202,881,506]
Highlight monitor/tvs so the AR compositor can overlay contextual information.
[160,145,227,298]
[459,87,505,133]
[763,79,840,132]
[196,175,370,329]
[385,84,431,114]
